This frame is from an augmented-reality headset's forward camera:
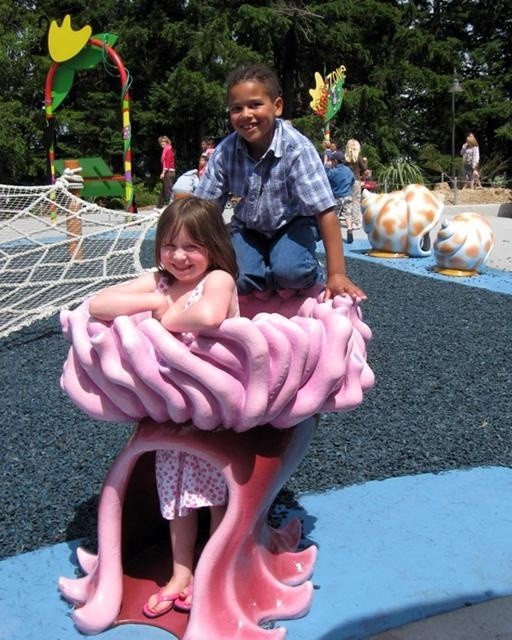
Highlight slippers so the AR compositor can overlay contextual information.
[143,584,194,617]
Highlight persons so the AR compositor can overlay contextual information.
[195,155,209,179]
[458,132,476,190]
[154,134,177,210]
[461,135,480,190]
[198,137,208,151]
[85,193,244,622]
[187,63,372,308]
[320,137,371,244]
[199,138,217,158]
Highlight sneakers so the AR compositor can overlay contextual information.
[347,229,353,243]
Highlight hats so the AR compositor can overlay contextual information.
[327,151,345,160]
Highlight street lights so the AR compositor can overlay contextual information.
[447,67,464,189]
